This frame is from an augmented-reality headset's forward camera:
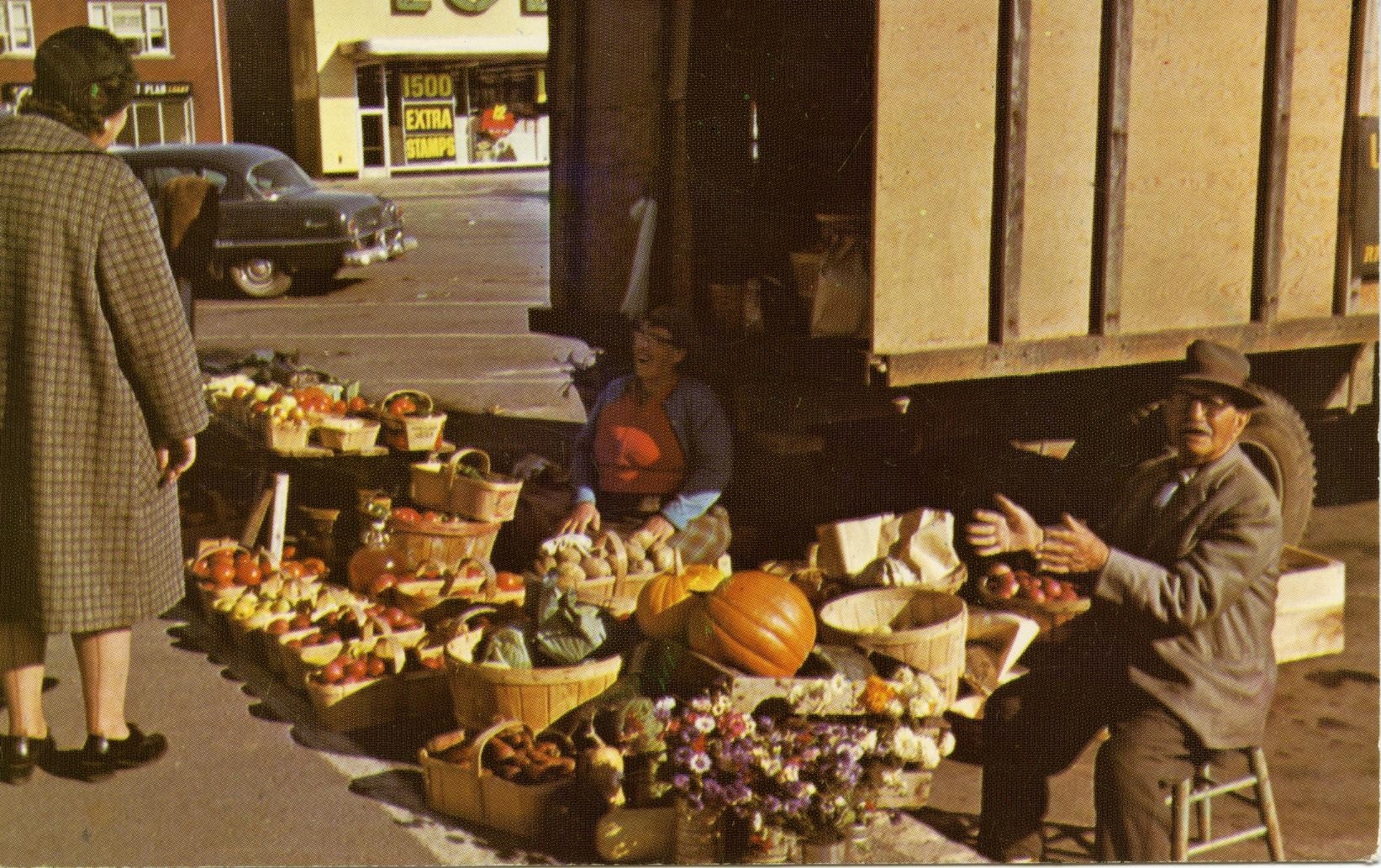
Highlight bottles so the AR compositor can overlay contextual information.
[349,518,404,593]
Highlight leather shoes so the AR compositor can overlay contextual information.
[3,726,57,785]
[77,723,168,783]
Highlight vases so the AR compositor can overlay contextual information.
[668,760,933,866]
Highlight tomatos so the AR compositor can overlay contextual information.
[191,381,524,690]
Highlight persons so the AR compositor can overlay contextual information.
[969,338,1284,864]
[500,306,733,565]
[0,25,209,784]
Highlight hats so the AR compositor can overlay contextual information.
[647,305,700,343]
[1166,340,1266,408]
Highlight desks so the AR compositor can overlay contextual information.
[207,413,459,567]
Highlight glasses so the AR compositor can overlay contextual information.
[1168,391,1231,409]
[634,328,680,346]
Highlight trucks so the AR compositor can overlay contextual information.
[520,4,1377,580]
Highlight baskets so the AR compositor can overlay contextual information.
[417,719,574,838]
[537,531,662,616]
[387,519,500,567]
[185,543,448,731]
[443,622,624,737]
[411,447,523,523]
[210,389,448,452]
[819,587,969,682]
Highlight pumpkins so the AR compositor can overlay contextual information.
[638,544,817,679]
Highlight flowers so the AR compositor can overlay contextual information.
[648,665,957,856]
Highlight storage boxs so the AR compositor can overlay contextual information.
[185,544,451,736]
[316,418,382,453]
[815,585,969,713]
[443,600,626,734]
[208,367,363,440]
[354,503,502,578]
[367,387,448,452]
[178,481,227,559]
[976,571,1094,643]
[442,620,624,736]
[1269,543,1347,666]
[524,527,684,630]
[260,421,308,453]
[417,720,586,840]
[393,557,527,634]
[296,503,340,539]
[406,447,524,524]
[658,639,843,715]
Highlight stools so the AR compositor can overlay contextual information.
[1167,746,1287,862]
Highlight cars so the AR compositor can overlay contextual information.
[105,146,407,301]
